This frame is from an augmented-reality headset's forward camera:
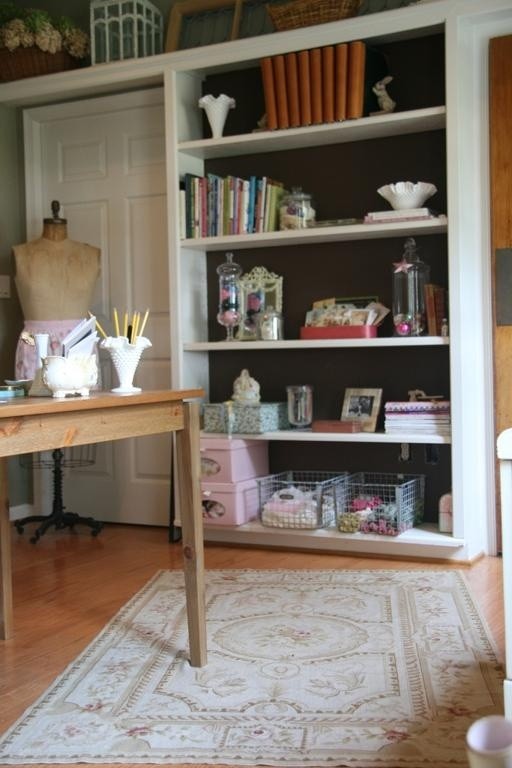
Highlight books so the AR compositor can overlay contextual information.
[180,173,290,239]
[384,400,452,434]
[58,316,99,358]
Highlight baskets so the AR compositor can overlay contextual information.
[331,471,427,536]
[255,470,350,530]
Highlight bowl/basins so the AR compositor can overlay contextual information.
[376,180,438,209]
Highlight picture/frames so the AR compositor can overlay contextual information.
[340,386,384,433]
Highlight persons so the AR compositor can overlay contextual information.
[9,214,103,390]
[349,395,371,416]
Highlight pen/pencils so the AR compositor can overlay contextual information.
[87,310,108,339]
[113,307,120,338]
[124,310,140,345]
[139,308,150,337]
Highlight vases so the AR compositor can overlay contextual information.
[27,334,52,397]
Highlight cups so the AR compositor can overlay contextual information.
[98,334,153,397]
[287,384,314,429]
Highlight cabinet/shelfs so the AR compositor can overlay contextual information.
[164,3,489,565]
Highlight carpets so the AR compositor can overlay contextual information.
[1,566,508,768]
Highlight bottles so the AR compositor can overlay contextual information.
[216,251,243,342]
[390,237,431,336]
[278,184,315,232]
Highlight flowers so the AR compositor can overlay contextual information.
[0,0,91,65]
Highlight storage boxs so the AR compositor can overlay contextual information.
[198,440,276,527]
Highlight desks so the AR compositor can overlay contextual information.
[0,386,210,669]
